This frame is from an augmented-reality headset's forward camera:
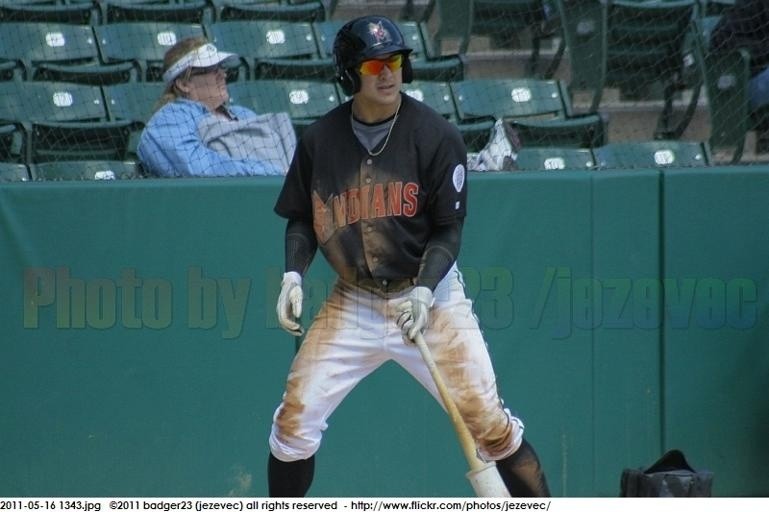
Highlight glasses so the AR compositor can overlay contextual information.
[189,63,227,79]
[358,55,404,79]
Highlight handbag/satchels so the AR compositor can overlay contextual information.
[619,450,713,498]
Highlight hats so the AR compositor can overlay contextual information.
[159,43,241,84]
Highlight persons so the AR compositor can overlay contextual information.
[135,38,283,177]
[261,16,551,497]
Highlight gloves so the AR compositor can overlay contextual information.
[275,270,306,337]
[388,285,437,342]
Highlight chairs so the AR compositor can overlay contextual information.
[0,0,769,182]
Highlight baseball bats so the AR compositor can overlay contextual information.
[413,330,510,498]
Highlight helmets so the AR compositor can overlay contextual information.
[331,15,413,70]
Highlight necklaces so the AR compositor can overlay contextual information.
[351,104,400,157]
